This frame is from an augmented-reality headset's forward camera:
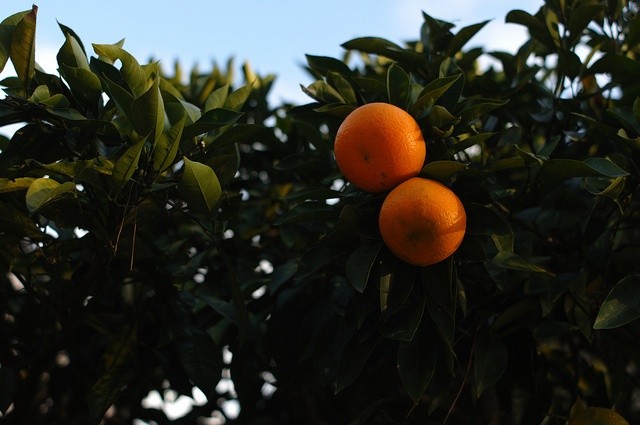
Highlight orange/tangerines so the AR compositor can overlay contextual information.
[334,101,427,190]
[378,178,467,264]
